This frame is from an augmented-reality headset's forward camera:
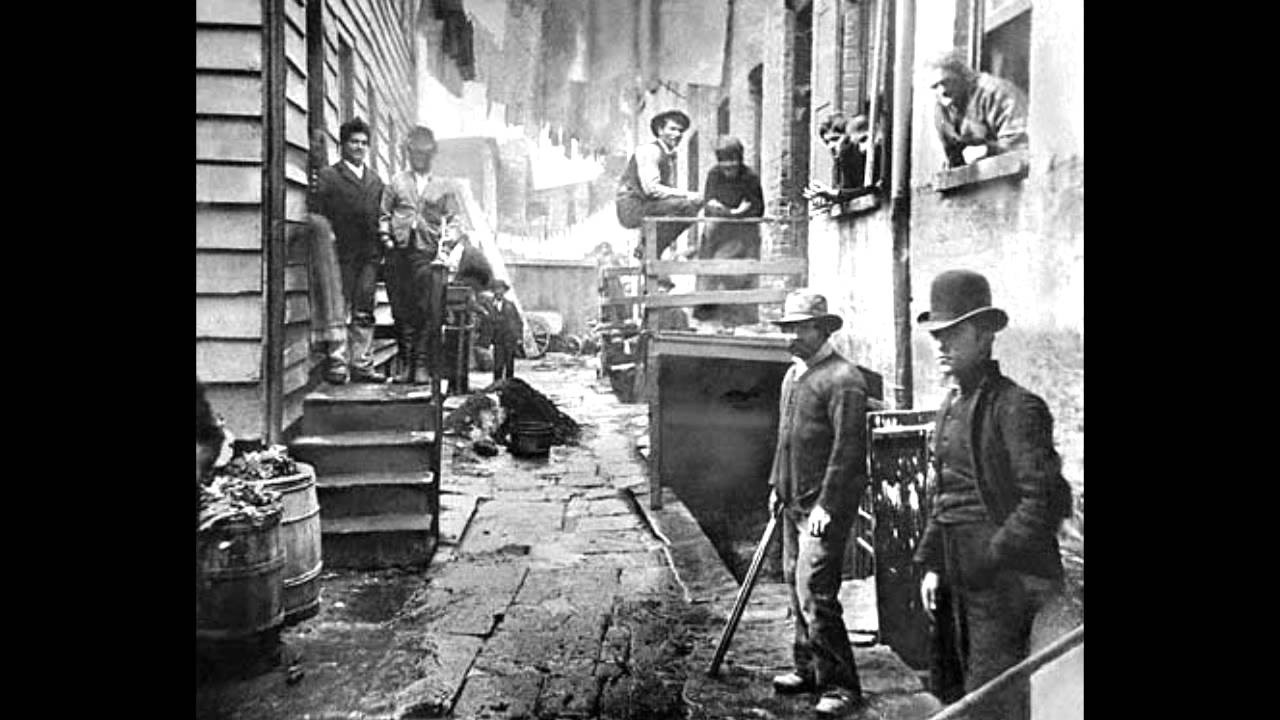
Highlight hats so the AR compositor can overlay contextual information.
[770,291,843,332]
[490,281,510,291]
[915,269,1009,332]
[650,108,690,141]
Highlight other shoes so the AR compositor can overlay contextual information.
[351,366,387,382]
[413,367,430,383]
[773,669,821,696]
[393,366,413,383]
[816,696,853,717]
[327,365,346,383]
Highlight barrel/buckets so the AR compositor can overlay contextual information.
[193,492,287,677]
[230,461,325,616]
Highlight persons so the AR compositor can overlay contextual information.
[915,270,1064,719]
[309,52,1034,390]
[767,287,870,718]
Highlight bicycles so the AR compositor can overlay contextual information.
[516,314,554,359]
